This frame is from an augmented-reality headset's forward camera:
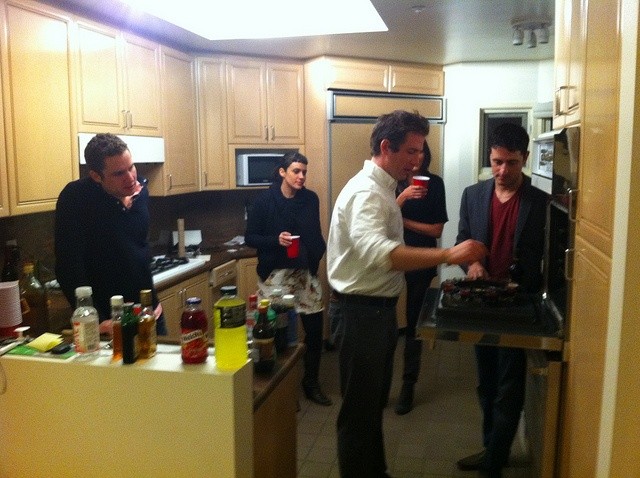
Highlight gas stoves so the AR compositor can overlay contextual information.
[44,247,188,291]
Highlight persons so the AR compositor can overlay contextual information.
[390,139,449,415]
[55,133,166,334]
[454,124,552,478]
[245,153,331,406]
[324,108,489,478]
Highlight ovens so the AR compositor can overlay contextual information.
[415,126,580,342]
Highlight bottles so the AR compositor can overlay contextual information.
[120,302,140,365]
[254,299,274,332]
[275,304,289,352]
[252,304,278,381]
[213,285,248,372]
[110,295,124,361]
[282,294,298,350]
[180,297,209,362]
[246,295,259,340]
[139,289,158,360]
[2,239,21,281]
[72,286,99,362]
[271,289,283,310]
[19,261,49,338]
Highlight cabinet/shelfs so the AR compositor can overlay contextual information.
[211,261,238,307]
[1,0,73,216]
[156,266,213,340]
[559,231,616,478]
[163,50,199,194]
[232,56,304,149]
[301,57,443,344]
[76,15,158,134]
[200,51,231,191]
[554,1,620,257]
[238,256,260,301]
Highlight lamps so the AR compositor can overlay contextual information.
[510,19,550,49]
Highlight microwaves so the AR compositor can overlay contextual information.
[237,154,287,187]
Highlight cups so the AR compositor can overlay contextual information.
[412,176,431,188]
[286,235,300,258]
[0,280,24,339]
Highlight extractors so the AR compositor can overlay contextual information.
[78,135,165,167]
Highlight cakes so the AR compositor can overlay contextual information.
[440,275,495,311]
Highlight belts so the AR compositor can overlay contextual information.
[332,290,399,306]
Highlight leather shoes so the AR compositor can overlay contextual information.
[306,390,333,407]
[397,381,414,415]
[456,448,509,469]
